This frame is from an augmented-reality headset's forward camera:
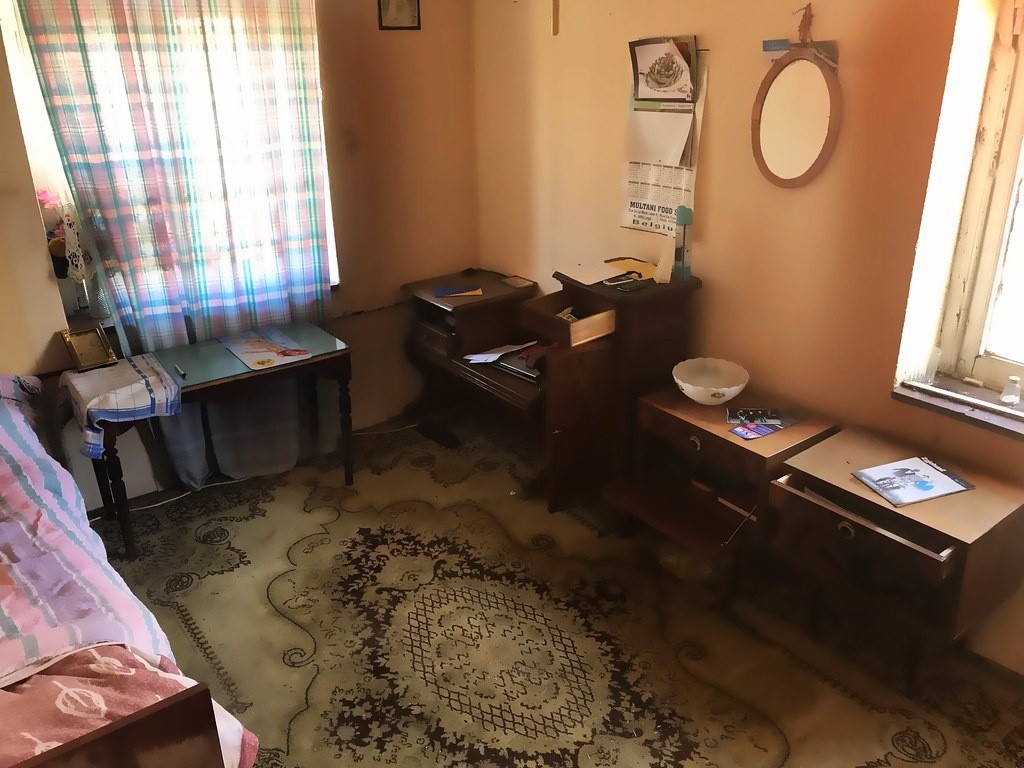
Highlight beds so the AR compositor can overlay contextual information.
[0,373,260,768]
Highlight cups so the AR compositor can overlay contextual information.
[909,345,942,385]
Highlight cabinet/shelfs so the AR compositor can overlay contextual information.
[398,259,706,500]
[770,423,1024,642]
[601,382,837,564]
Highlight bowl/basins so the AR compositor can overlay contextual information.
[672,358,749,406]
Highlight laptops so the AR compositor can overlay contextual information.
[495,352,541,380]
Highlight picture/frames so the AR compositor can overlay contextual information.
[58,321,119,372]
[377,0,421,30]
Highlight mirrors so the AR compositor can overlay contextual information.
[751,47,841,188]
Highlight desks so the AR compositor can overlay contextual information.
[62,317,355,562]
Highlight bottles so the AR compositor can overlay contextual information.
[1000,376,1021,407]
